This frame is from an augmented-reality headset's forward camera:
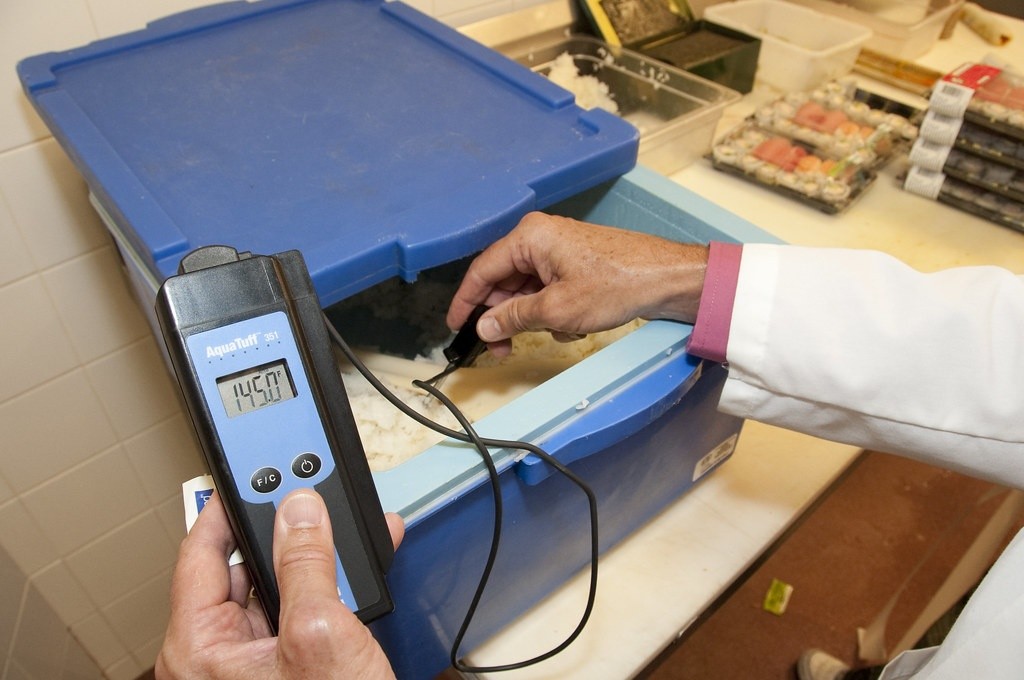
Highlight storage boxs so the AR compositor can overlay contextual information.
[13,0,966,680]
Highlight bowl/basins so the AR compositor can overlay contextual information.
[800,0,966,60]
[514,37,743,177]
[703,0,872,93]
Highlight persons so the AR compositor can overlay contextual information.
[150,209,1024,680]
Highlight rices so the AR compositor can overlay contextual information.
[530,45,671,116]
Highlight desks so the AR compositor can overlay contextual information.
[438,85,1024,680]
